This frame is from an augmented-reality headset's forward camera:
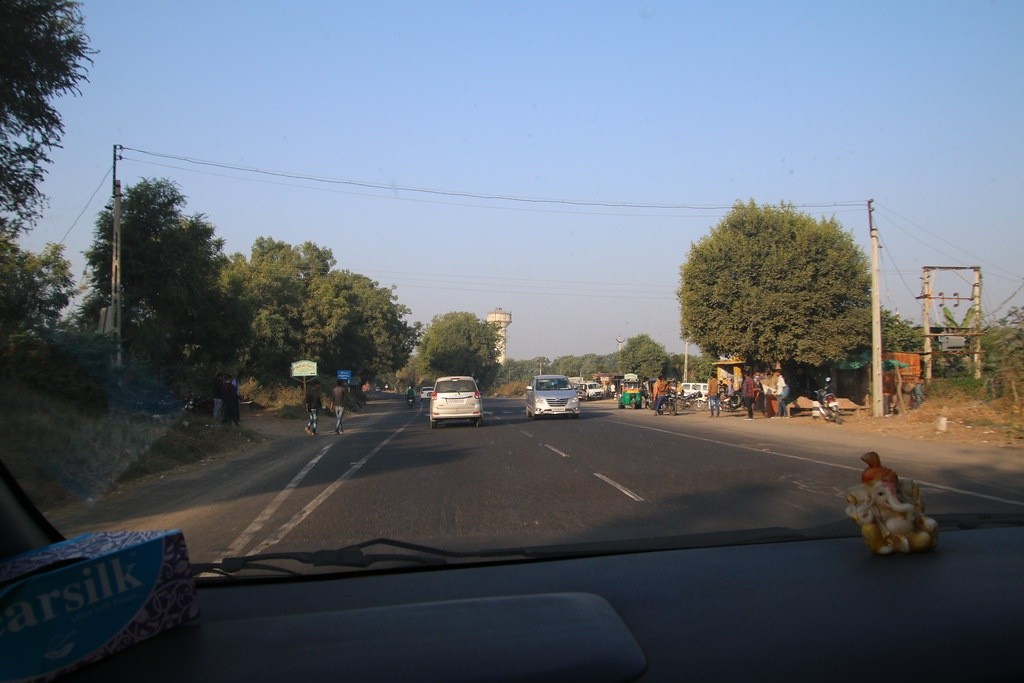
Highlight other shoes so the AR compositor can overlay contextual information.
[304,427,311,433]
[771,415,781,418]
[311,429,315,435]
[744,418,753,420]
[654,411,657,416]
[340,432,343,434]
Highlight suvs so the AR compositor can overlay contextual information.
[430,375,484,428]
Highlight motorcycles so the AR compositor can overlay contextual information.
[657,390,677,415]
[619,375,642,409]
[675,388,705,411]
[721,389,746,412]
[813,376,840,424]
[407,395,415,408]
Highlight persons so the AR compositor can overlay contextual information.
[330,378,346,434]
[719,365,790,418]
[652,376,673,416]
[406,386,417,405]
[706,372,721,418]
[304,380,321,435]
[611,384,615,399]
[742,371,755,418]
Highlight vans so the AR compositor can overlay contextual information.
[376,387,380,392]
[681,383,708,397]
[582,383,604,400]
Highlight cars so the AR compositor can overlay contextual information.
[420,387,434,401]
[525,375,581,420]
[571,384,585,401]
